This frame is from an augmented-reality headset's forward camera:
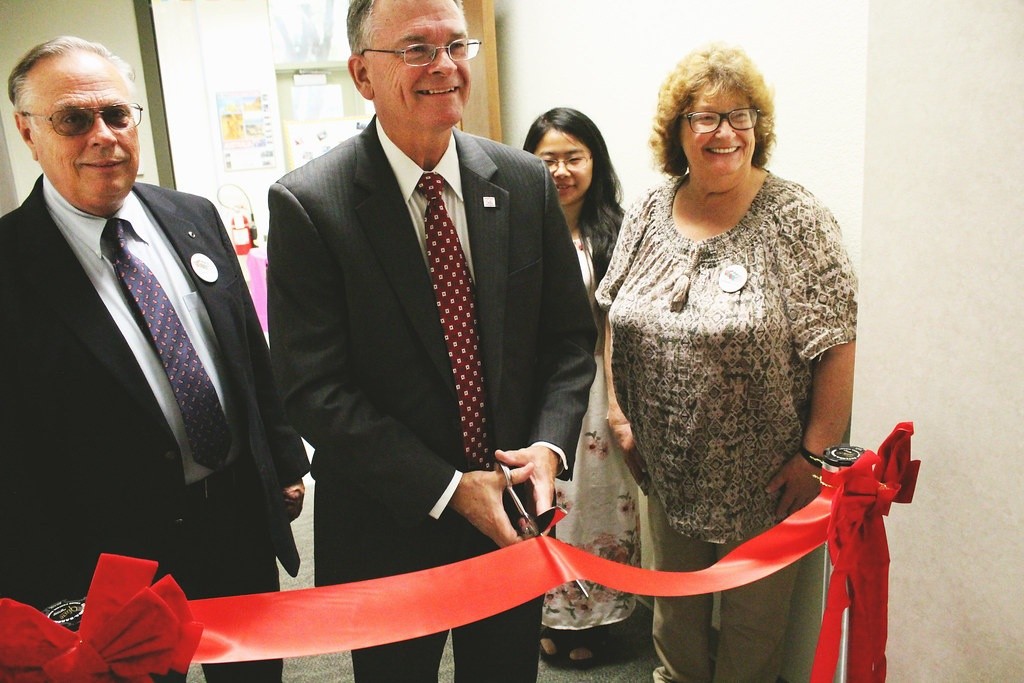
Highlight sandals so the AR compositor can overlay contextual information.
[540,624,571,659]
[569,625,610,670]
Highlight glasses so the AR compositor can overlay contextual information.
[542,156,594,174]
[21,104,144,137]
[361,37,482,66]
[681,107,760,134]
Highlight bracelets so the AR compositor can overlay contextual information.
[800,445,822,468]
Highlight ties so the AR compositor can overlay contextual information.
[413,173,492,472]
[100,217,232,473]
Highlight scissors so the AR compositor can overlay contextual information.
[493,459,594,604]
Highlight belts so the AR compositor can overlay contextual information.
[179,465,248,507]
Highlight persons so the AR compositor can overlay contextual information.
[522,109,640,669]
[594,47,859,683]
[0,38,310,683]
[266,1,595,683]
